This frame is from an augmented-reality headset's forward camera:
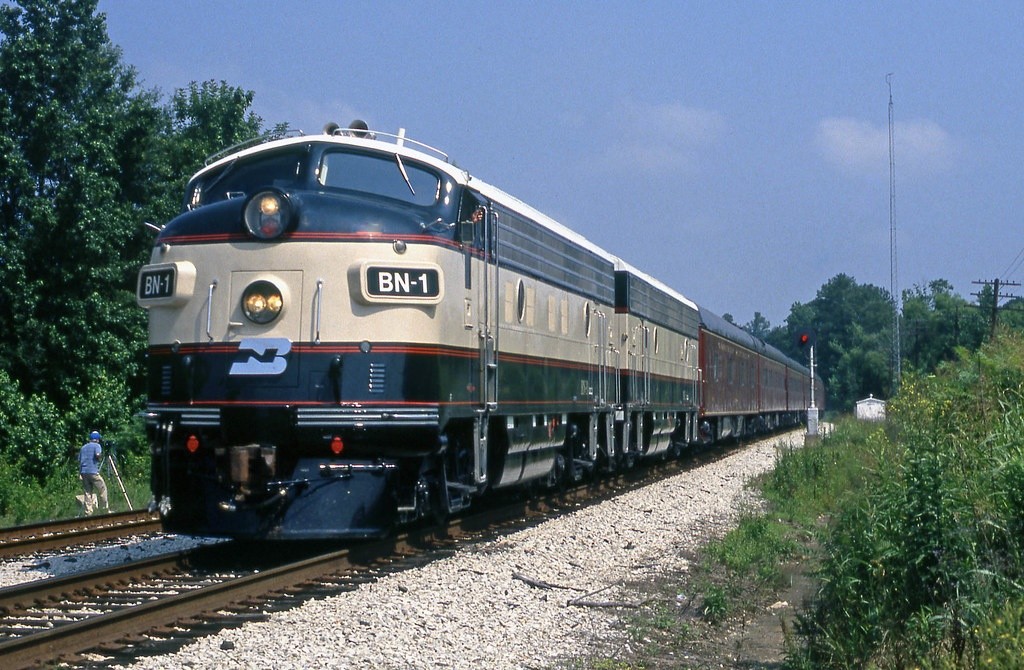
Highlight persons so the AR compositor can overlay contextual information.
[80,431,115,516]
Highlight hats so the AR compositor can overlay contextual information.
[88,431,103,439]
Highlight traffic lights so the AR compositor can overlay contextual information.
[797,328,813,351]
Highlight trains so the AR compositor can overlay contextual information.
[138,121,826,546]
